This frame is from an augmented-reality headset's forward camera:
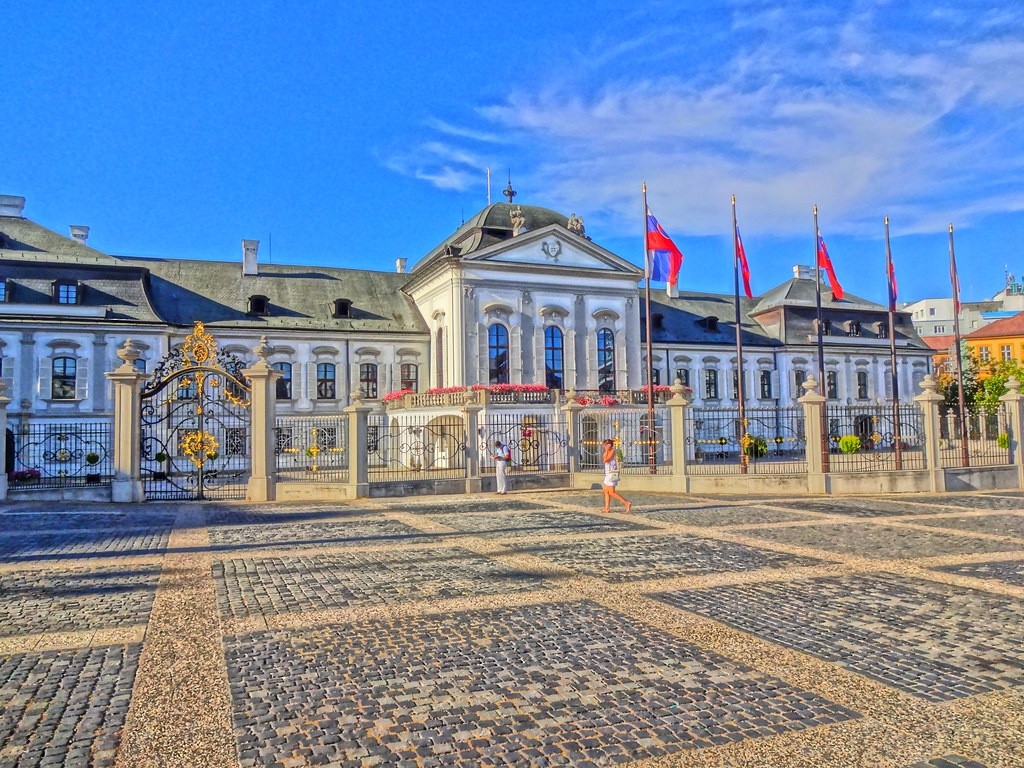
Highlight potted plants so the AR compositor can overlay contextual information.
[831,434,842,455]
[504,461,512,474]
[522,440,529,463]
[695,450,704,464]
[617,451,624,469]
[303,447,315,475]
[717,437,728,459]
[773,436,784,456]
[87,452,101,484]
[206,450,219,479]
[153,453,166,481]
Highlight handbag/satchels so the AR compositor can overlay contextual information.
[609,470,620,482]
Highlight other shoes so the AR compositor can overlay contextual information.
[600,509,610,513]
[625,502,631,513]
[500,492,507,495]
[495,492,501,494]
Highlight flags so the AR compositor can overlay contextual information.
[817,225,844,299]
[735,219,753,300]
[646,204,683,287]
[886,232,898,313]
[949,240,960,314]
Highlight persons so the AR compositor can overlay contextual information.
[600,439,631,513]
[493,441,509,495]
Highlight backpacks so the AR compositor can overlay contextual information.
[502,445,511,461]
